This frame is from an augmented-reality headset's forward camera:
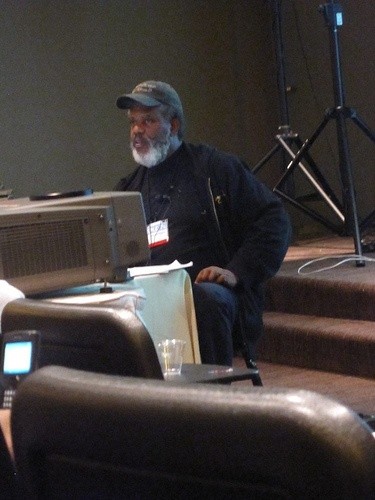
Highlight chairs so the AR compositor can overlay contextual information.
[0,298,166,385]
[10,367,375,500]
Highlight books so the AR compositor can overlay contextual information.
[126,259,193,280]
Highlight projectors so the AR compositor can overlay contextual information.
[0,188,152,298]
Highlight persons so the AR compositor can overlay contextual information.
[116,79,292,385]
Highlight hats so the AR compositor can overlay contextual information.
[116,80,182,111]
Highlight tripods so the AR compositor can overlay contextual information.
[247,0,375,266]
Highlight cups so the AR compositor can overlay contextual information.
[156,339,187,376]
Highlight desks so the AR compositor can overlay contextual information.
[162,362,264,386]
[40,267,202,366]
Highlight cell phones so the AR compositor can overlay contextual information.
[0,330,41,410]
